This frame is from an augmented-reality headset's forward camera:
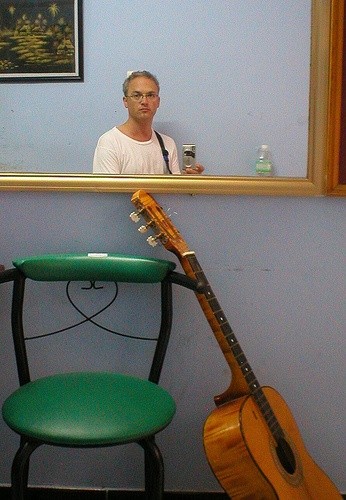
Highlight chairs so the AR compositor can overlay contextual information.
[0,253,206,500]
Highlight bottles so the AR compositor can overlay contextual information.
[255,144,272,177]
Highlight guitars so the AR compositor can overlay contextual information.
[128,189,343,500]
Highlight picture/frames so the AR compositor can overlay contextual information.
[0,0,84,83]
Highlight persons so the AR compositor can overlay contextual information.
[93,71,204,175]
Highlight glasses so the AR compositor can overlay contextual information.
[125,93,159,102]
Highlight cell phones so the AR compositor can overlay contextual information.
[182,144,196,170]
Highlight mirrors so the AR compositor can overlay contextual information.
[0,0,331,197]
